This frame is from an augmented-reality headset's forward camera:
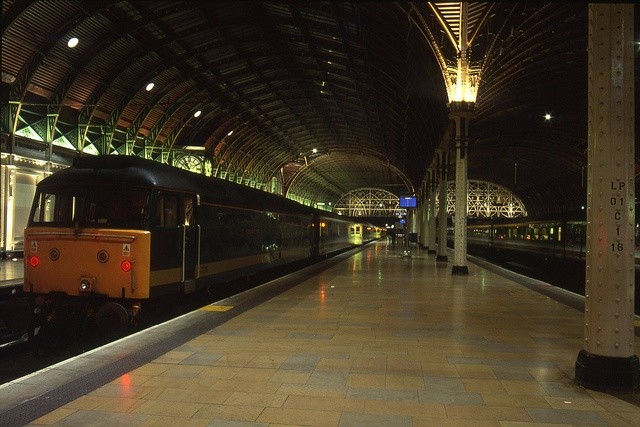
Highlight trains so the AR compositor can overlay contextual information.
[21,155,388,326]
